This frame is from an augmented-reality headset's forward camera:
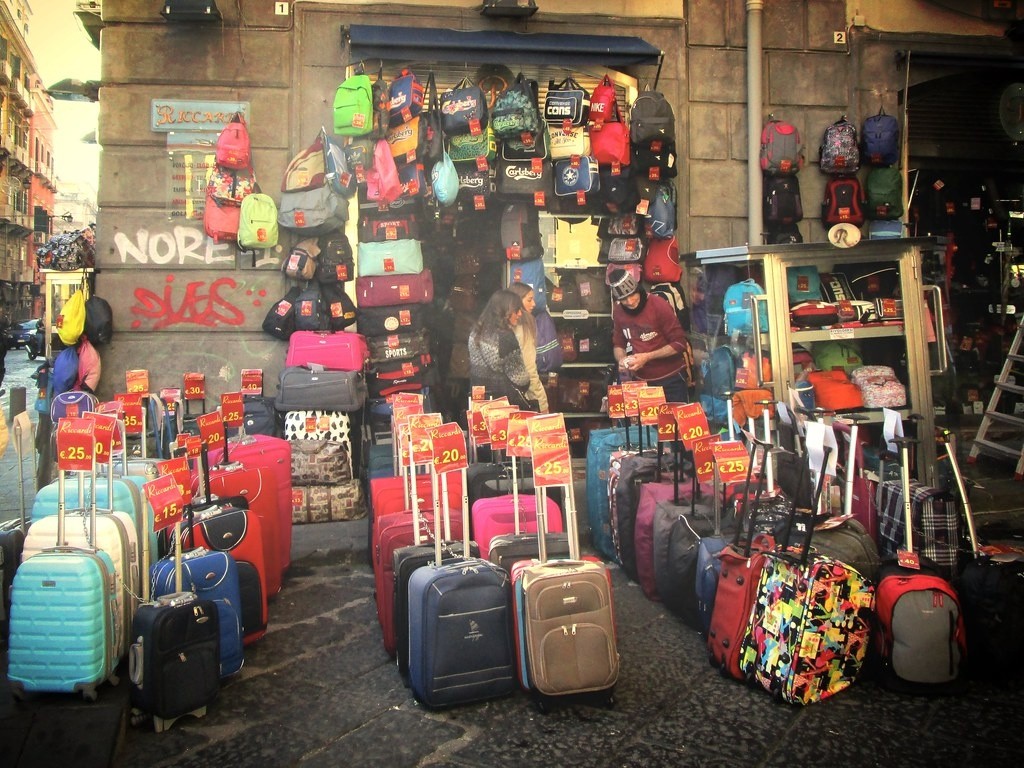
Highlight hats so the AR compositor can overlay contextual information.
[606,268,640,301]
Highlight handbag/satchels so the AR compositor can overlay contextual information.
[506,377,535,407]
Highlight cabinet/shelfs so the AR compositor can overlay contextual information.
[691,238,947,490]
[504,212,610,421]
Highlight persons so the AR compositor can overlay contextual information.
[507,282,550,413]
[36,318,45,353]
[610,269,689,404]
[469,282,531,410]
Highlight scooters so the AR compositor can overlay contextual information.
[24,332,46,360]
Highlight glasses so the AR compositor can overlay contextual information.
[511,306,520,314]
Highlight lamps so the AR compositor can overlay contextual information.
[160,0,220,24]
[81,129,97,144]
[46,75,100,103]
[473,0,539,18]
[73,11,104,49]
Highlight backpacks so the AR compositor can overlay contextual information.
[27,67,1015,362]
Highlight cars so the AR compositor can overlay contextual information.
[7,318,40,350]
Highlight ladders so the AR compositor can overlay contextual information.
[967,312,1024,483]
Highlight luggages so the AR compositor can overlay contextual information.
[1,363,1024,730]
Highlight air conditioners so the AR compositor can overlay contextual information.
[34,231,46,246]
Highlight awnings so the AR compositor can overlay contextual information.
[348,24,666,90]
